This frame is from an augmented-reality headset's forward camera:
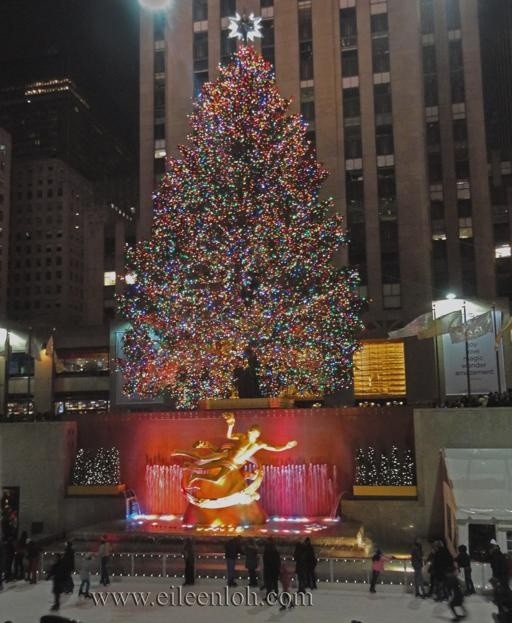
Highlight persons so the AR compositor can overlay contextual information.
[15,529,29,579]
[1,532,18,583]
[222,532,317,610]
[171,410,299,509]
[98,532,112,583]
[412,534,511,622]
[368,545,395,593]
[45,552,68,611]
[181,537,195,585]
[77,551,97,598]
[63,541,76,594]
[25,537,41,585]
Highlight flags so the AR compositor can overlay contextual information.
[448,310,492,342]
[45,333,55,356]
[493,313,511,349]
[416,308,462,340]
[388,311,432,339]
[25,333,42,361]
[4,333,13,360]
